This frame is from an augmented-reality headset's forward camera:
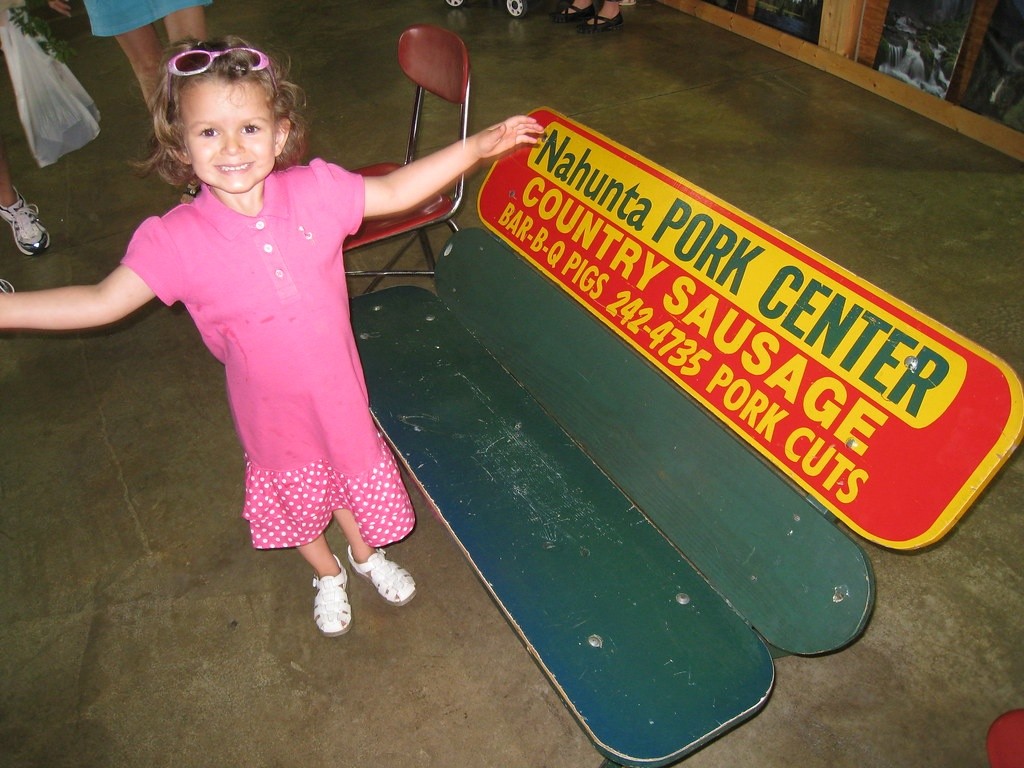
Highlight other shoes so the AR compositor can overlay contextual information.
[575,11,624,34]
[618,0,636,6]
[552,2,596,23]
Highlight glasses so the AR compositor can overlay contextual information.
[167,48,279,101]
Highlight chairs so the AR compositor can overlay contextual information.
[345,26,469,297]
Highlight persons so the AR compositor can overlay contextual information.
[0,0,214,294]
[0,35,546,638]
[549,0,623,33]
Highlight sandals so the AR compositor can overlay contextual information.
[312,553,353,638]
[180,181,200,205]
[347,543,417,607]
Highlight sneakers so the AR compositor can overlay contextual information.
[0,185,50,255]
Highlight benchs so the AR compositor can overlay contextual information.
[350,108,1024,768]
